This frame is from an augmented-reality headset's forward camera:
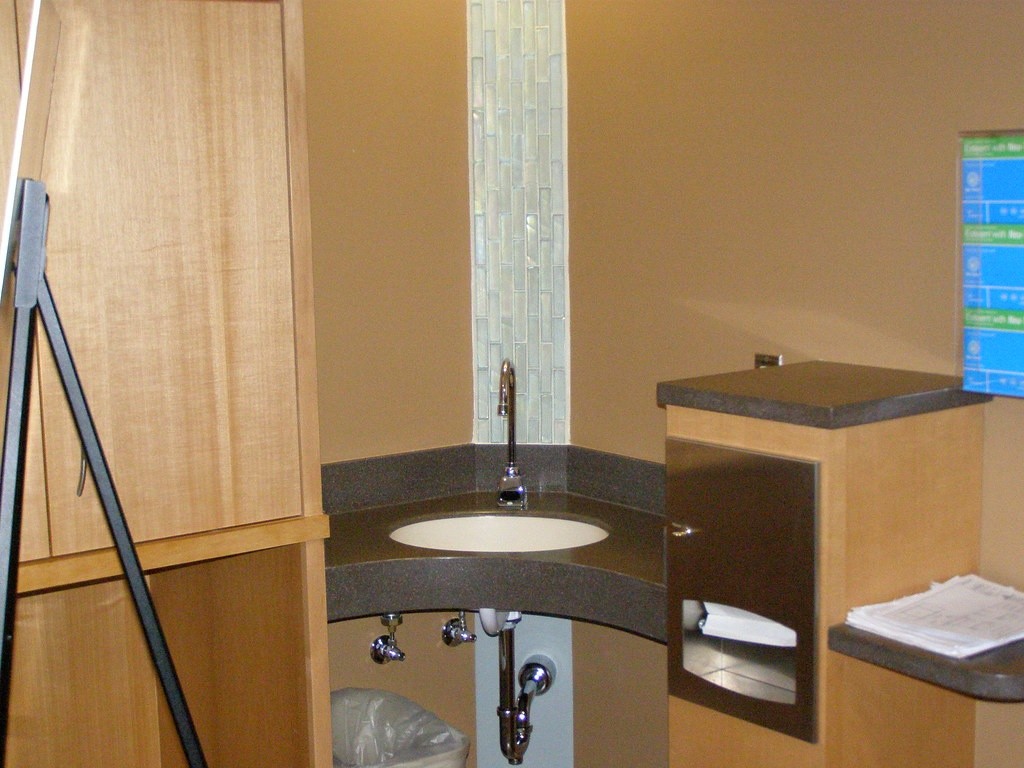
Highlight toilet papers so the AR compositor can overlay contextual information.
[702,602,797,647]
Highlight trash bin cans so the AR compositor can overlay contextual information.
[330,687,471,768]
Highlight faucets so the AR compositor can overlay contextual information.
[496,358,528,507]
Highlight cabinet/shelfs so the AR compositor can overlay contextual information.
[0,1,331,768]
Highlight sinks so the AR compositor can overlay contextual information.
[386,511,613,553]
